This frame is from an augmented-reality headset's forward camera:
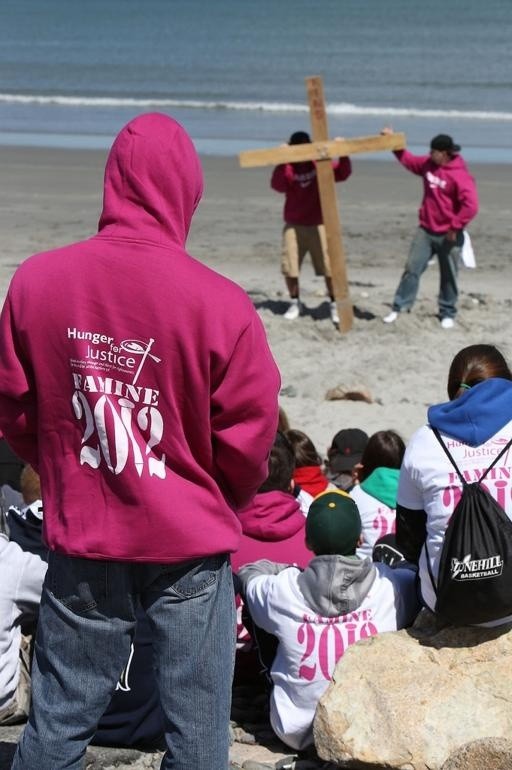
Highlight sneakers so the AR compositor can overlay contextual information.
[329,302,340,323]
[283,299,303,319]
[382,312,398,323]
[441,317,454,328]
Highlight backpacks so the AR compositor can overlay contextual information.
[424,424,512,624]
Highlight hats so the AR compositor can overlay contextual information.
[290,132,310,144]
[430,134,461,152]
[328,428,369,473]
[307,489,362,555]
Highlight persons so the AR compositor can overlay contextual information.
[399,344,512,634]
[5,463,45,560]
[0,529,50,727]
[0,113,282,770]
[276,404,291,436]
[229,439,317,573]
[0,442,26,534]
[323,428,370,491]
[236,493,404,753]
[380,125,479,330]
[286,429,351,516]
[349,431,407,561]
[270,131,353,326]
